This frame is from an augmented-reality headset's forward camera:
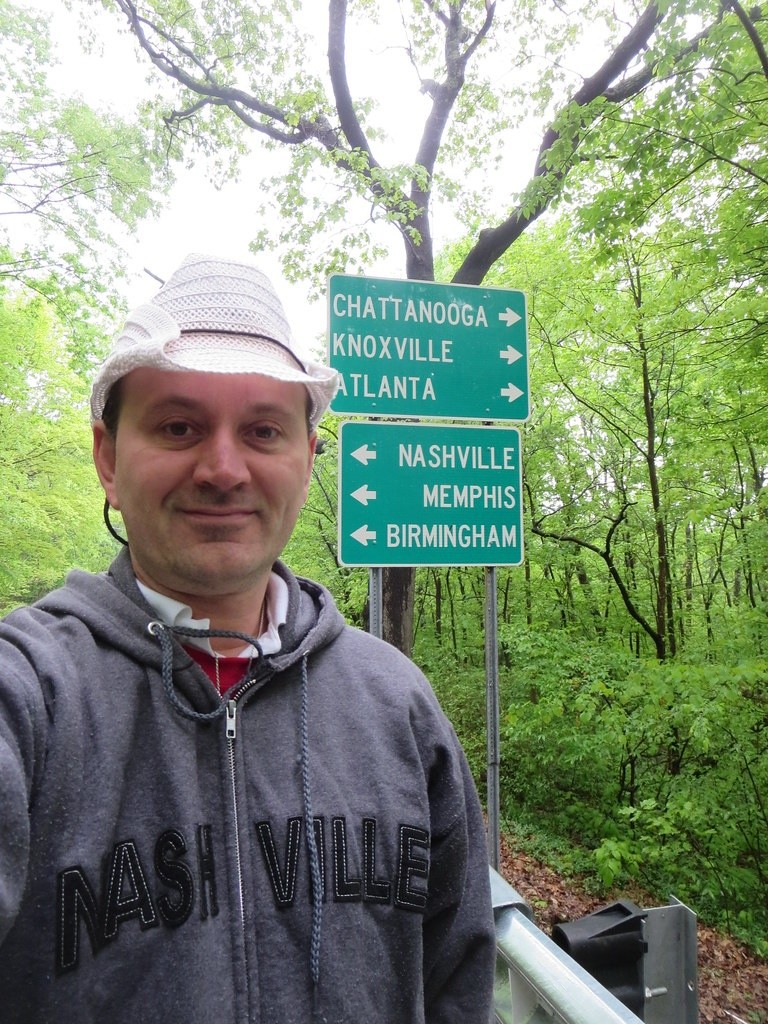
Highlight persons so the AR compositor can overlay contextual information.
[0,252,498,1024]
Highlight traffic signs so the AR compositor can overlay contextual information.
[335,421,525,569]
[325,272,532,423]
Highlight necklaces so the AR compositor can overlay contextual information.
[213,604,264,692]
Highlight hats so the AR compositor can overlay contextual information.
[90,253,339,443]
